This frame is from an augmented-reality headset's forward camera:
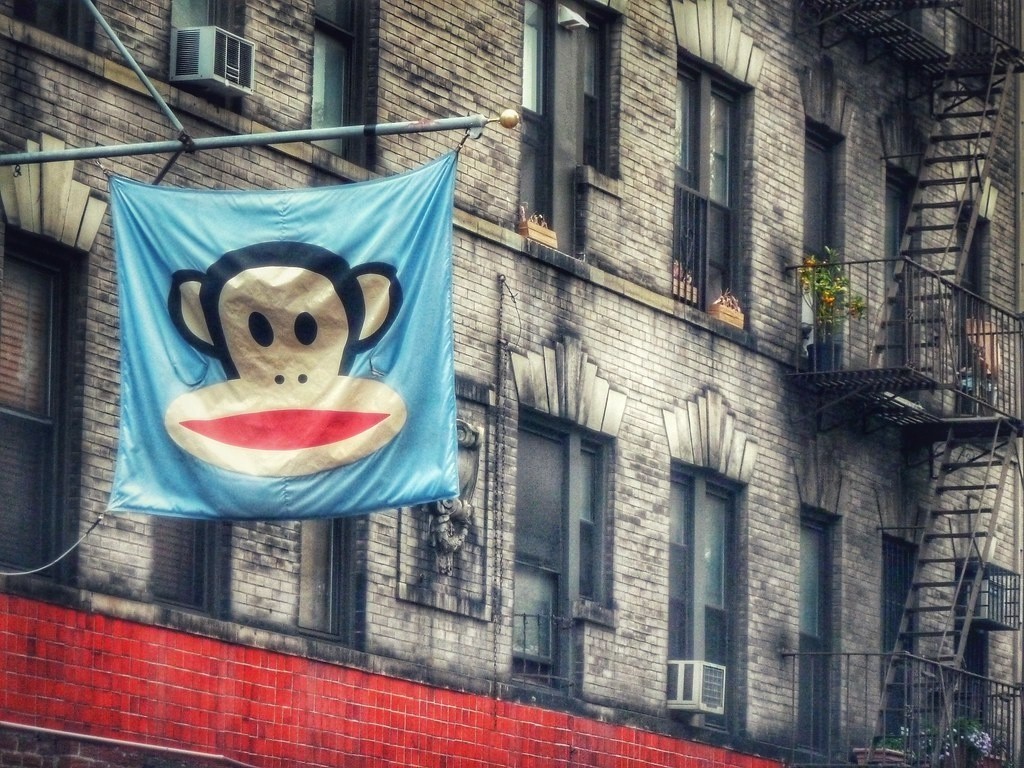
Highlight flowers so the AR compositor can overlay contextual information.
[672,259,692,286]
[797,245,867,342]
[876,715,1006,768]
[712,288,745,313]
[518,201,548,229]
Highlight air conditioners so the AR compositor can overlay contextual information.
[170,25,257,97]
[667,659,727,716]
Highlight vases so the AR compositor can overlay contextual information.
[807,343,845,371]
[673,278,698,304]
[707,304,744,330]
[977,754,1004,768]
[520,221,559,248]
[853,747,912,765]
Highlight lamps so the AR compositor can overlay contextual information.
[558,3,590,32]
